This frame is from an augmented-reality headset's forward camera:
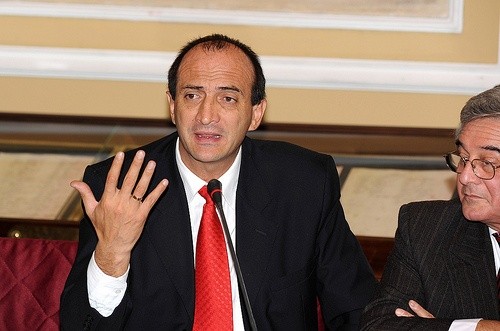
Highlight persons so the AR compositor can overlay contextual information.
[361,85,500,331]
[58,33,381,331]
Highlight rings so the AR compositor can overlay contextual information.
[132,194,143,203]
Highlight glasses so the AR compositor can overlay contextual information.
[443,150,500,180]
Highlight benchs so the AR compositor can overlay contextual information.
[0,217,500,331]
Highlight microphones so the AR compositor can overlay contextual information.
[207,179,258,331]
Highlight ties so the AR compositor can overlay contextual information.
[192,185,235,331]
